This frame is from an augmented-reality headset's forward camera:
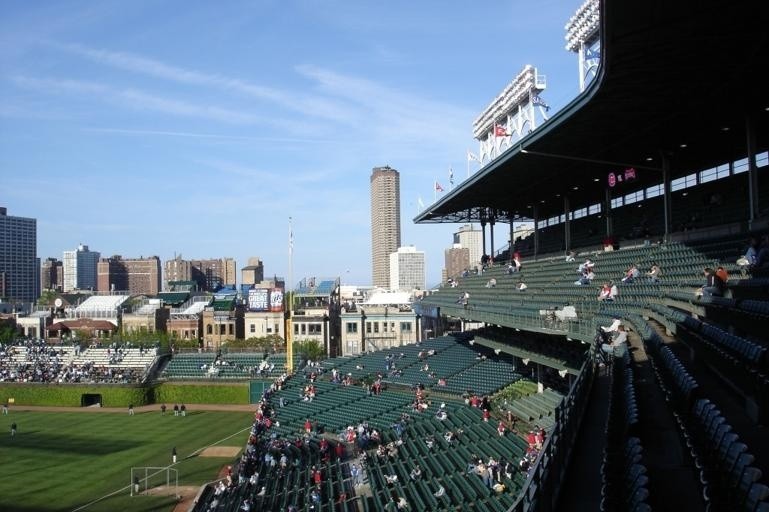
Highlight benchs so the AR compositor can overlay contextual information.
[160,171,769,512]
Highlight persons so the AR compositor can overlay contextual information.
[0,233,760,510]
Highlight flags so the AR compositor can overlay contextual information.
[417,43,597,209]
